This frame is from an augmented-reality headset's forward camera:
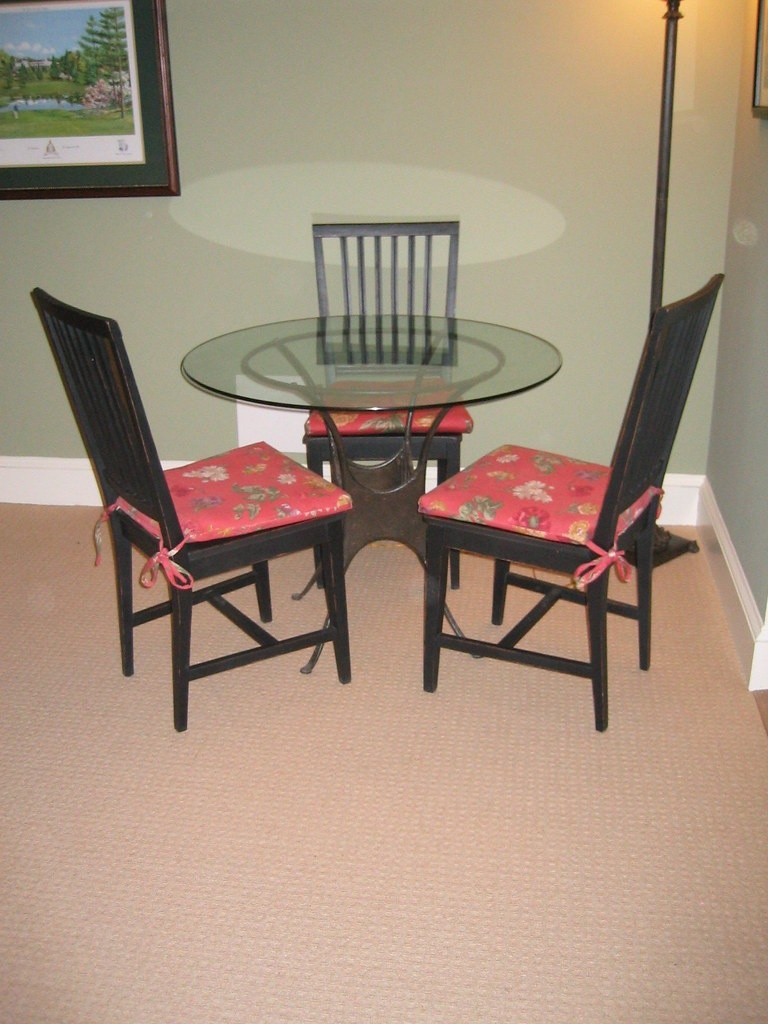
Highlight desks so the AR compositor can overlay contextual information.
[179,315,562,673]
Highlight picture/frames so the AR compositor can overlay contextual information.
[753,0,768,109]
[0,0,181,201]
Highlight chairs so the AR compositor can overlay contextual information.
[293,220,476,589]
[30,286,356,732]
[417,272,726,732]
